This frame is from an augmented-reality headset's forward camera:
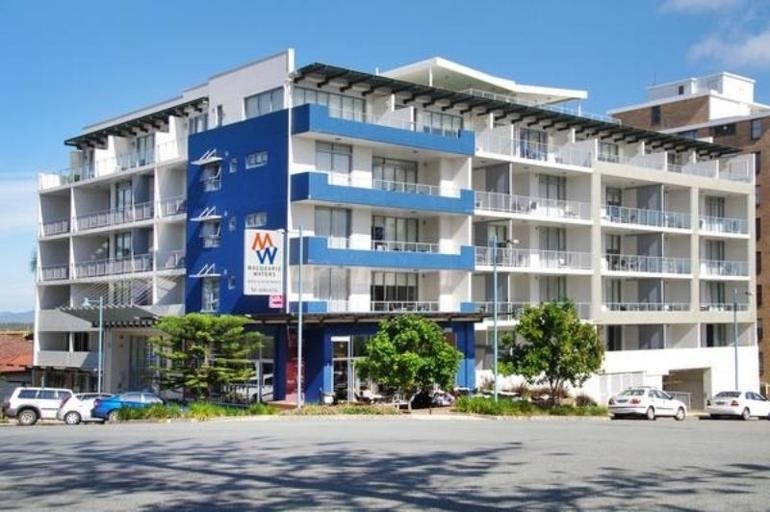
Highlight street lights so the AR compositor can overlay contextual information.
[81,296,104,392]
[492,234,520,403]
[734,286,752,389]
[276,224,304,409]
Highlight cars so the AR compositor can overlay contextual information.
[225,373,274,403]
[2,385,76,426]
[706,390,770,420]
[91,391,166,421]
[56,392,113,425]
[607,383,688,421]
